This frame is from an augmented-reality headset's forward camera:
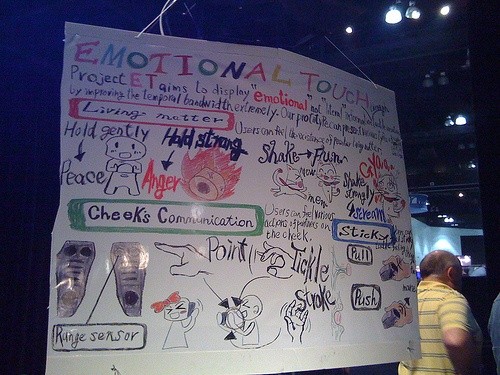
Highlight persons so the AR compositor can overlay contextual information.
[398,249,485,375]
[487,293,500,375]
